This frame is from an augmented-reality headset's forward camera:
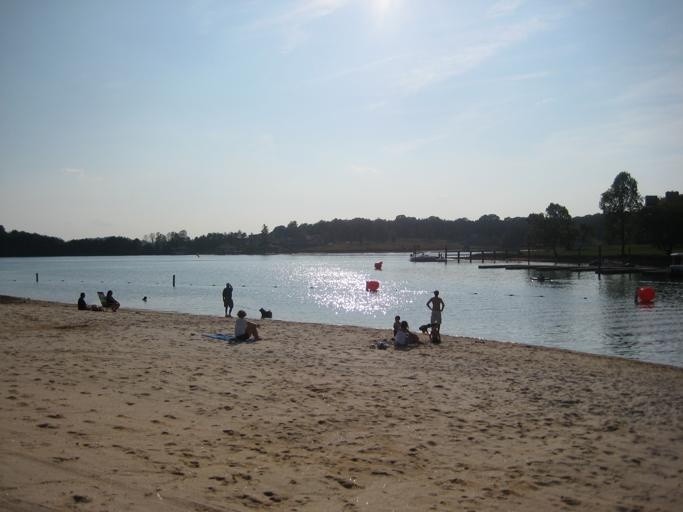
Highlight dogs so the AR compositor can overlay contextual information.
[259,308,272,319]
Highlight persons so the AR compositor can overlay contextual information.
[427,290,444,334]
[421,251,444,259]
[429,326,441,342]
[634,286,640,304]
[78,292,90,311]
[222,283,234,316]
[234,311,262,342]
[393,315,400,336]
[105,290,120,311]
[396,321,411,351]
[143,295,148,301]
[419,323,431,334]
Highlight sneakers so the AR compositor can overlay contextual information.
[255,338,262,340]
[225,315,231,317]
